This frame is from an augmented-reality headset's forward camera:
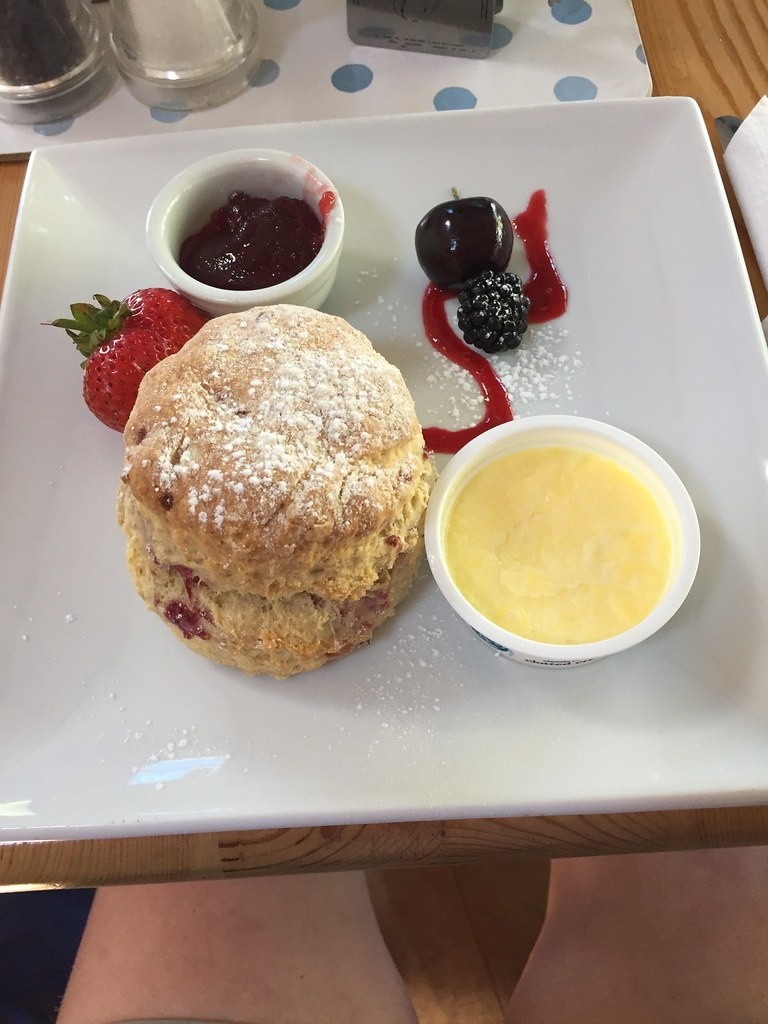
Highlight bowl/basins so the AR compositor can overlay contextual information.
[429,416,703,670]
[144,147,347,318]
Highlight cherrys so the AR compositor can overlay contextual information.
[415,189,513,291]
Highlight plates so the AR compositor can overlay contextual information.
[0,96,768,842]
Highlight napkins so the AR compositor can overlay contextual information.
[722,95,768,295]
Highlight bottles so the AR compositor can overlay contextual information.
[106,0,261,105]
[0,0,113,124]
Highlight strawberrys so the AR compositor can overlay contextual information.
[43,287,207,432]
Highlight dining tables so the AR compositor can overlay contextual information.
[0,1,768,1024]
